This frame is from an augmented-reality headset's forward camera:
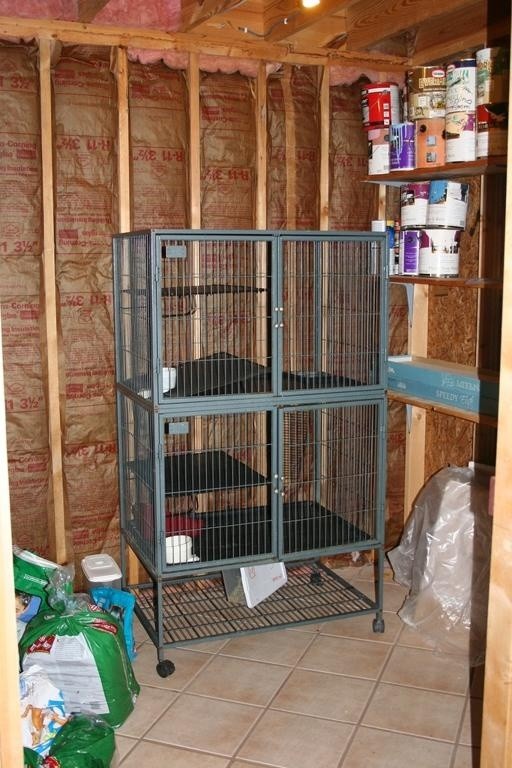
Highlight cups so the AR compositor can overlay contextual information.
[162,367,176,390]
[165,536,192,564]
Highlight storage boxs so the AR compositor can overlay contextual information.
[388,354,480,414]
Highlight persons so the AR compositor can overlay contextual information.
[15,590,43,645]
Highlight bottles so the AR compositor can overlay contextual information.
[385,220,395,275]
[392,221,401,275]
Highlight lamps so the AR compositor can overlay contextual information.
[238,1,321,38]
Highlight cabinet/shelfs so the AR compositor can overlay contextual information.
[114,230,387,676]
[363,160,499,425]
